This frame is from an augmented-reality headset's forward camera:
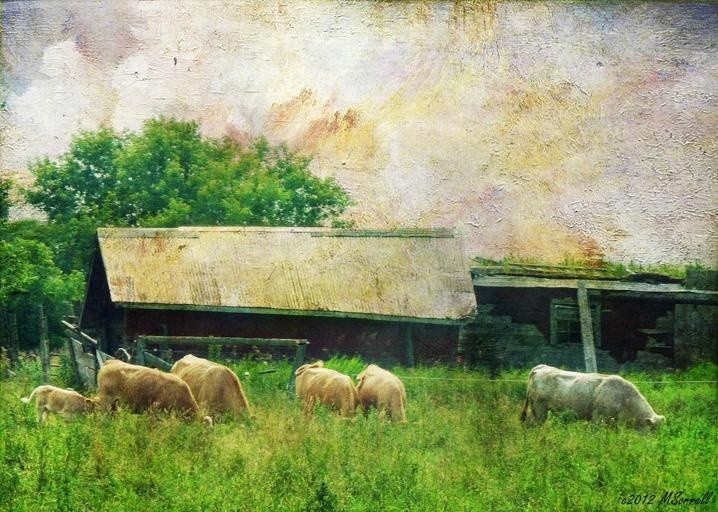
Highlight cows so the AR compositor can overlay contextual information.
[294,360,359,418]
[97,360,212,427]
[170,354,256,422]
[355,364,406,423]
[20,385,109,422]
[519,364,666,433]
